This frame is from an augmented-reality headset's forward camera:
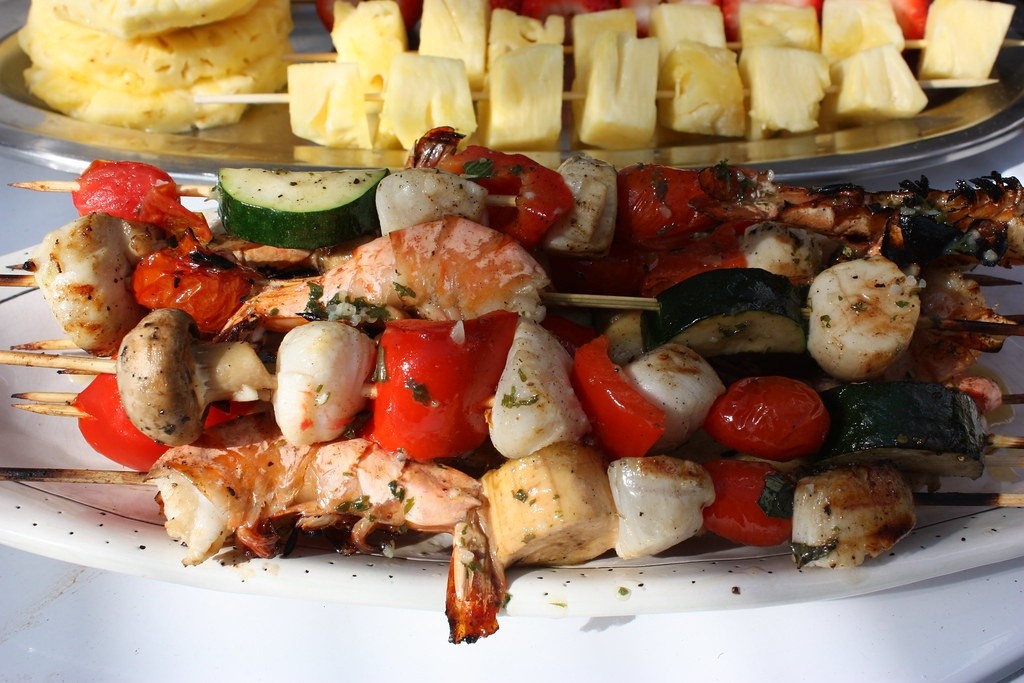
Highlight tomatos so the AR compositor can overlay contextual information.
[697,375,828,546]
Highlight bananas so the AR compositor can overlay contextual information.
[807,258,921,380]
[532,152,618,255]
[790,464,919,569]
[478,442,619,571]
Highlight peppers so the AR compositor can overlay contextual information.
[59,142,745,459]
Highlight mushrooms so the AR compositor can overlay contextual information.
[114,305,278,448]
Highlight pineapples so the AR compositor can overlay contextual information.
[22,0,1014,149]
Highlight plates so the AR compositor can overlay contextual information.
[3,14,1024,184]
[0,229,1023,617]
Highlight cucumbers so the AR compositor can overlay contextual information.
[218,164,392,251]
[818,380,985,480]
[635,267,808,358]
